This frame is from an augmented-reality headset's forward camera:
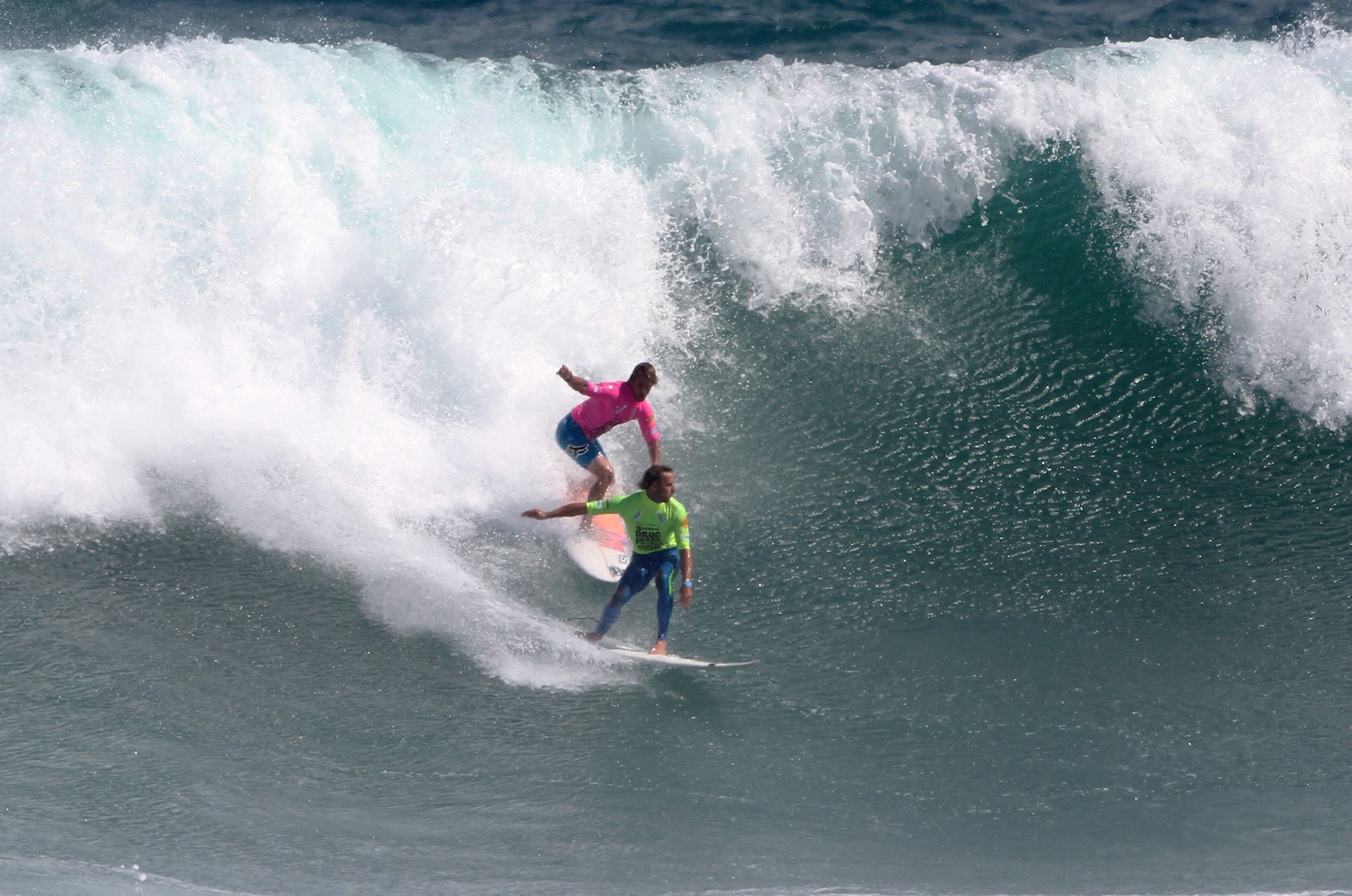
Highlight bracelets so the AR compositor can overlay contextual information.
[681,580,691,587]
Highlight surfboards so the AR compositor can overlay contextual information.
[547,463,630,590]
[587,635,764,671]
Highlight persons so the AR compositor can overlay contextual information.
[554,361,660,540]
[521,466,693,655]
